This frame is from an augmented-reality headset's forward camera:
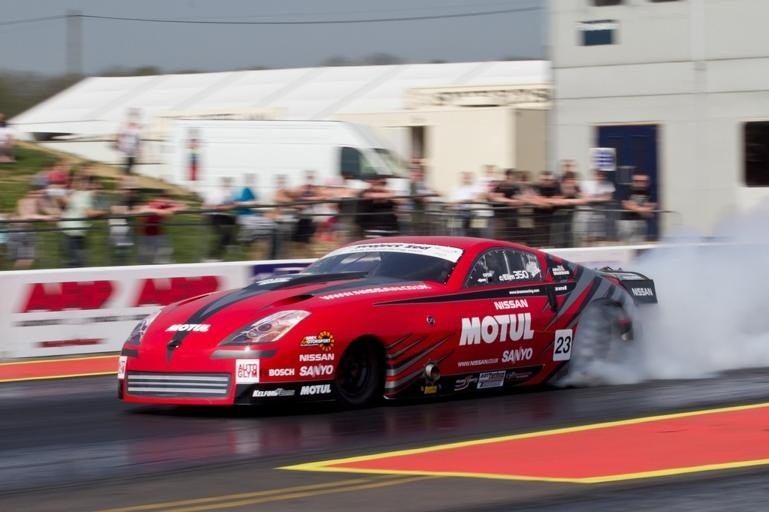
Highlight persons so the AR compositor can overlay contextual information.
[111,116,140,176]
[0,111,17,164]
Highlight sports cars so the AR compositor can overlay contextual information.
[115,233,658,410]
